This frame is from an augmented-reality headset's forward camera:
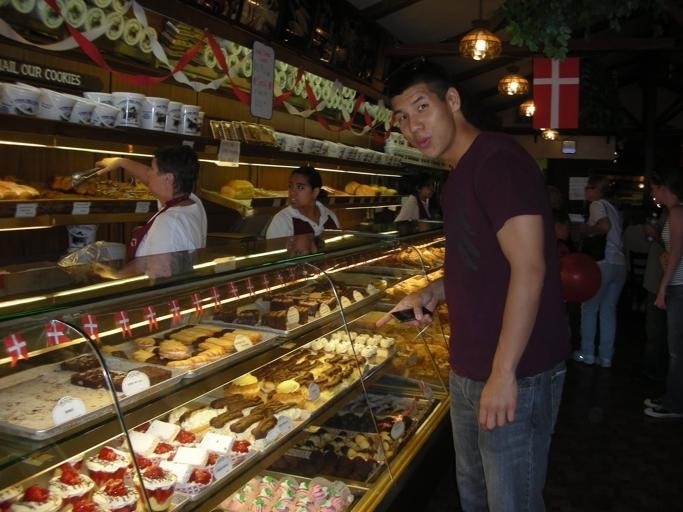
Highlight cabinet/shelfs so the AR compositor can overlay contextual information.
[0,115,451,512]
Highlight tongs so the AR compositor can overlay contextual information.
[72,167,101,187]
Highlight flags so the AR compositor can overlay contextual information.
[532,54,580,130]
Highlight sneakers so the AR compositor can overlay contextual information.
[644,404,683,418]
[644,397,663,407]
[574,350,594,364]
[596,355,613,367]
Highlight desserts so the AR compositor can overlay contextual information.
[0,238,443,512]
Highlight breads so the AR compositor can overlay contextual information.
[220,178,254,199]
[345,181,398,197]
[0,175,157,204]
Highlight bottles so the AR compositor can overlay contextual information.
[646,213,658,243]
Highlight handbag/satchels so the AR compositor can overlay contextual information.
[581,233,606,261]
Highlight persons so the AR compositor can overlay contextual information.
[263,164,340,241]
[83,143,208,262]
[94,248,186,279]
[285,231,324,259]
[392,172,437,221]
[381,56,573,510]
[531,163,683,421]
[410,220,433,232]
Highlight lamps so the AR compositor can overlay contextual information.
[453,0,560,142]
